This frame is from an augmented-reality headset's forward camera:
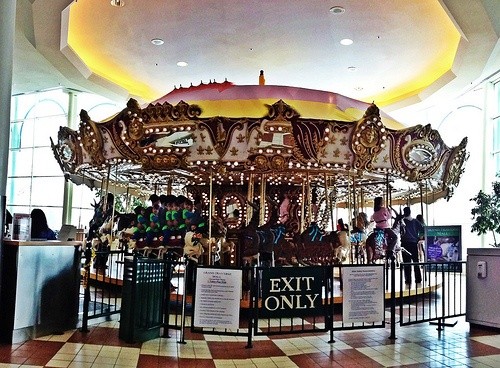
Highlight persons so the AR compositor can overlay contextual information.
[136,193,205,242]
[352,210,365,229]
[30,208,57,240]
[337,218,347,231]
[373,197,398,261]
[403,214,424,288]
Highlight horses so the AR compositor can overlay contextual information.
[88,198,413,265]
